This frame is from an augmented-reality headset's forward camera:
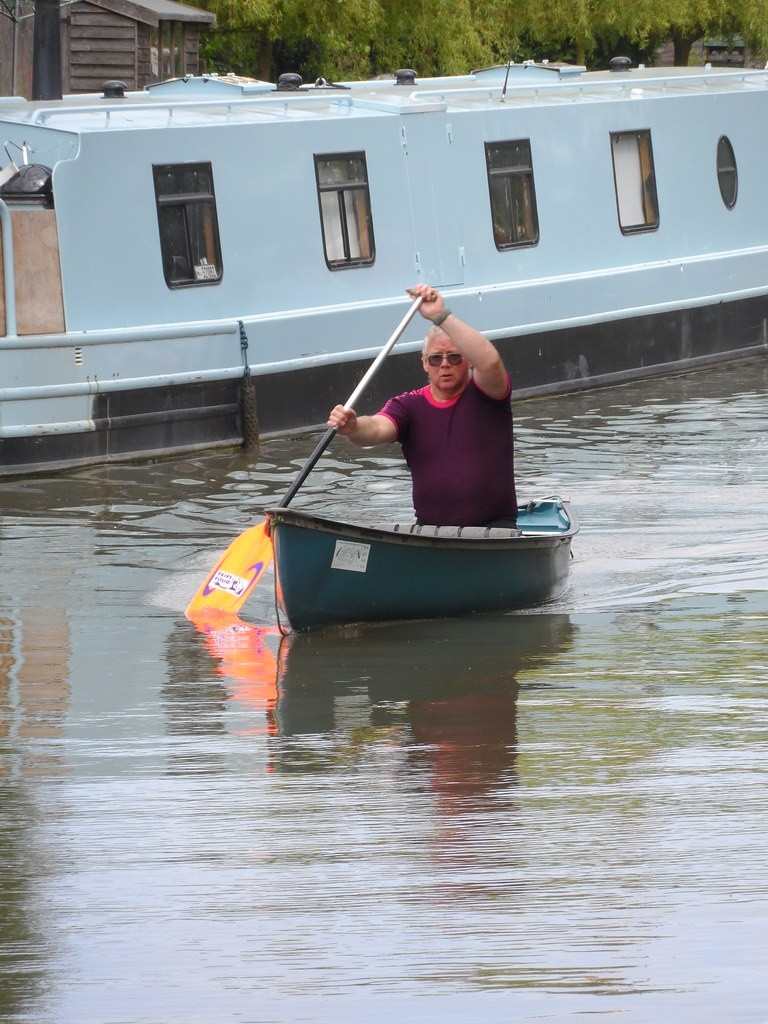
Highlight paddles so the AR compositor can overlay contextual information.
[184,288,437,615]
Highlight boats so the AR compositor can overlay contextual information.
[260,491,582,637]
[0,52,768,477]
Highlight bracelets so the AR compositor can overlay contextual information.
[434,308,451,325]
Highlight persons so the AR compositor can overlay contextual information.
[327,284,518,528]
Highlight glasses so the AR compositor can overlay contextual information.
[425,353,463,366]
